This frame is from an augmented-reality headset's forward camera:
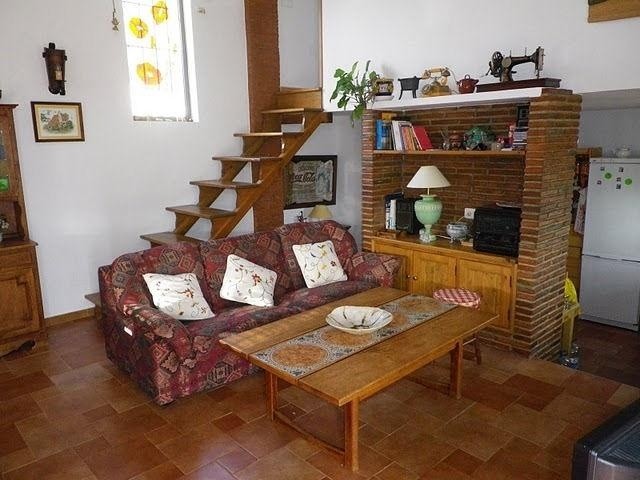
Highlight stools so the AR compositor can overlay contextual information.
[433,288,481,363]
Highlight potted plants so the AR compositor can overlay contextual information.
[329,61,383,127]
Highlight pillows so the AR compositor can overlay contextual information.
[141,273,216,320]
[292,240,348,288]
[221,253,278,308]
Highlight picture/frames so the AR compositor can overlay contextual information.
[31,101,85,142]
[283,155,338,209]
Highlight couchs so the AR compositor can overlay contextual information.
[97,220,404,408]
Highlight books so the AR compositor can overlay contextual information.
[376,112,433,150]
[385,192,418,235]
[512,126,527,151]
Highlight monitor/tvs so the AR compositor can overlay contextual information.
[572,398,639,480]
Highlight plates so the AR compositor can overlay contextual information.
[375,95,393,101]
[325,305,393,335]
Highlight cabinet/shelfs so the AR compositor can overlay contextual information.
[0,104,49,358]
[566,147,602,301]
[372,231,518,331]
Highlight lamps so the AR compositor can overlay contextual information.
[309,205,332,222]
[407,166,451,241]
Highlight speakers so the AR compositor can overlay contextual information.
[396,198,425,234]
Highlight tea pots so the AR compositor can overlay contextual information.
[456,75,479,94]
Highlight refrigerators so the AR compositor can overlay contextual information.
[577,156,640,332]
